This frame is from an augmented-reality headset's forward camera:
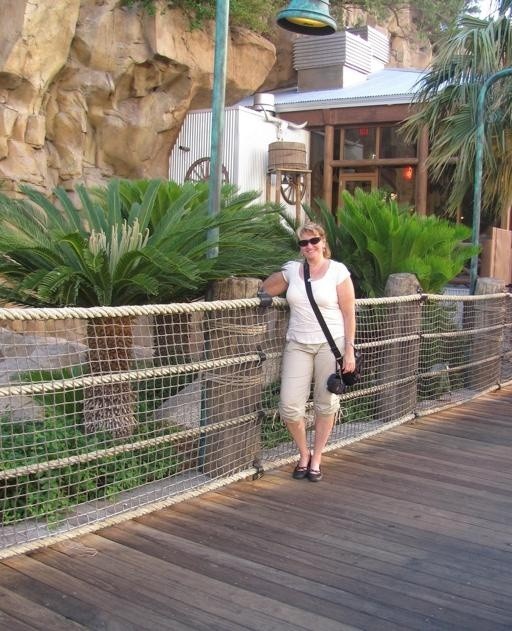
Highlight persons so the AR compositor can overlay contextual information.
[262,220,357,481]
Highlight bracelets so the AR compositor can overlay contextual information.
[346,342,355,348]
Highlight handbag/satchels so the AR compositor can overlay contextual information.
[338,349,362,386]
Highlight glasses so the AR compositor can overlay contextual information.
[297,236,322,247]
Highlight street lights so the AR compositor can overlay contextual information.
[205,1,337,264]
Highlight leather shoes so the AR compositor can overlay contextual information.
[293,451,312,479]
[309,461,323,482]
